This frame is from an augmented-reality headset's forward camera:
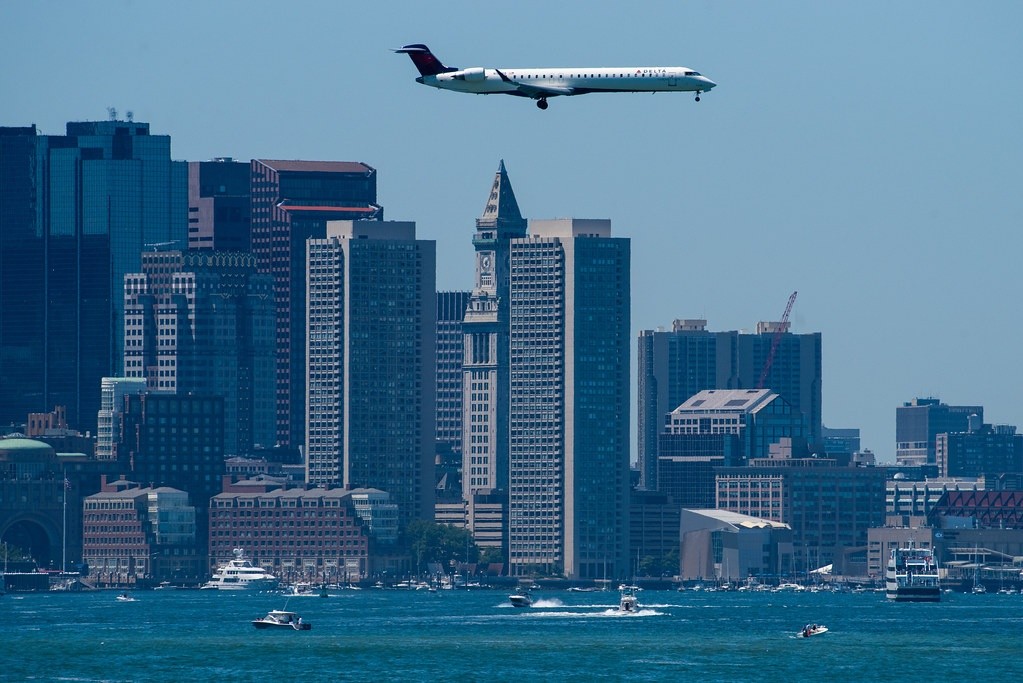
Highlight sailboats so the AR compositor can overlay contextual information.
[999,547,1007,594]
[570,548,645,592]
[971,541,987,595]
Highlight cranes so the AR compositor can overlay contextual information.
[757,291,799,391]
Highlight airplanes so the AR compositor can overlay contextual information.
[387,42,718,110]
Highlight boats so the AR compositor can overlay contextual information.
[201,548,282,591]
[510,590,535,608]
[886,537,942,603]
[1010,584,1018,593]
[677,549,887,595]
[250,610,311,631]
[154,581,177,592]
[516,578,542,592]
[116,596,140,603]
[797,625,829,639]
[282,574,476,596]
[617,590,640,615]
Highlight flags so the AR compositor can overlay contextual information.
[65,478,71,489]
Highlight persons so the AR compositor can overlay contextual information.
[805,624,817,637]
